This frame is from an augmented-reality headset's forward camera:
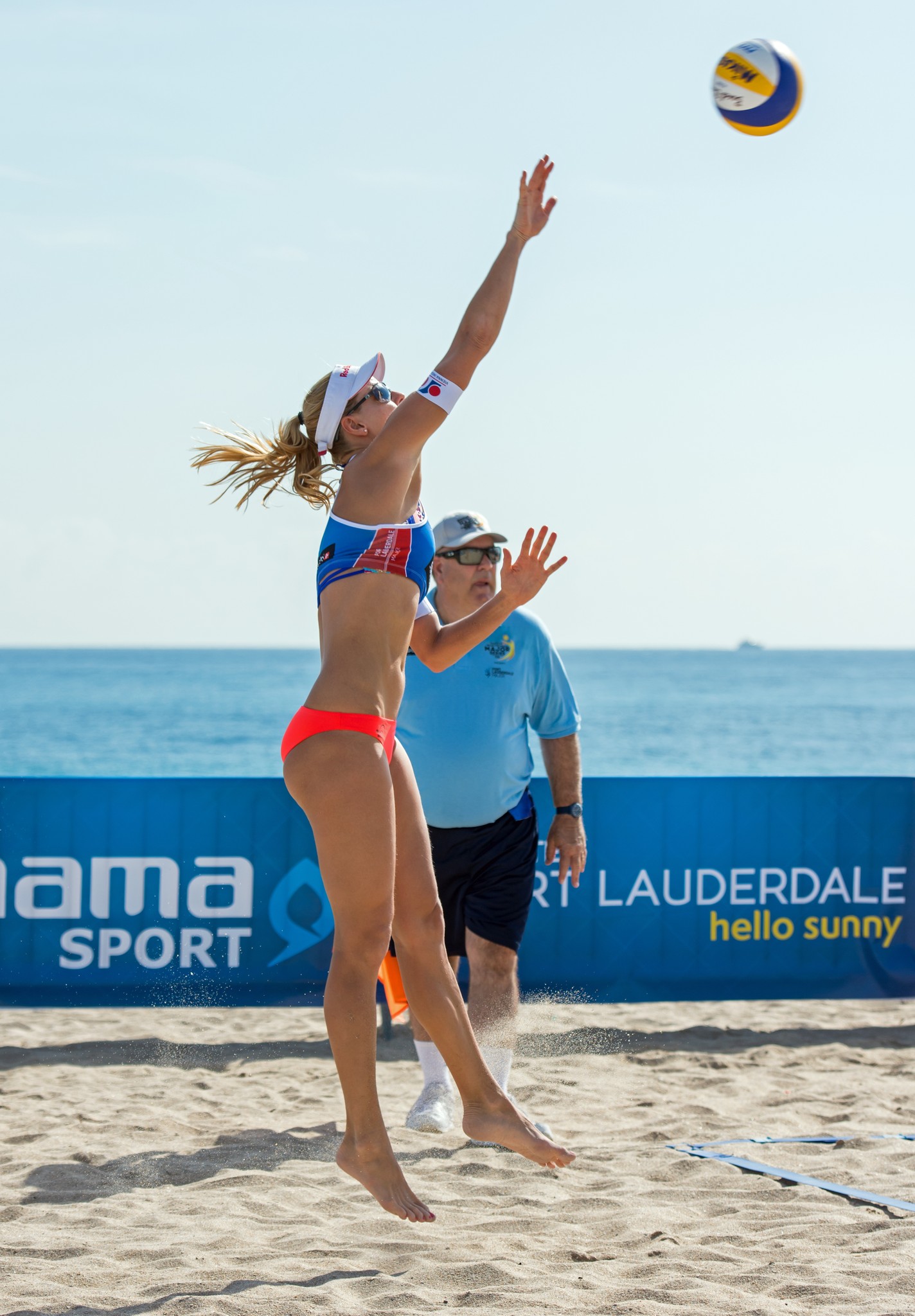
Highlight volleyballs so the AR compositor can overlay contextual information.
[713,38,803,137]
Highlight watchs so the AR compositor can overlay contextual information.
[555,801,583,818]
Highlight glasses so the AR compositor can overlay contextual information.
[335,382,391,443]
[435,546,501,565]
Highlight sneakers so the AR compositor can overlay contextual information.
[405,1082,456,1133]
[470,1093,553,1148]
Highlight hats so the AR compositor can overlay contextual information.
[315,351,385,456]
[432,512,508,552]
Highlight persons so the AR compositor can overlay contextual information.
[190,154,576,1223]
[398,512,588,1147]
